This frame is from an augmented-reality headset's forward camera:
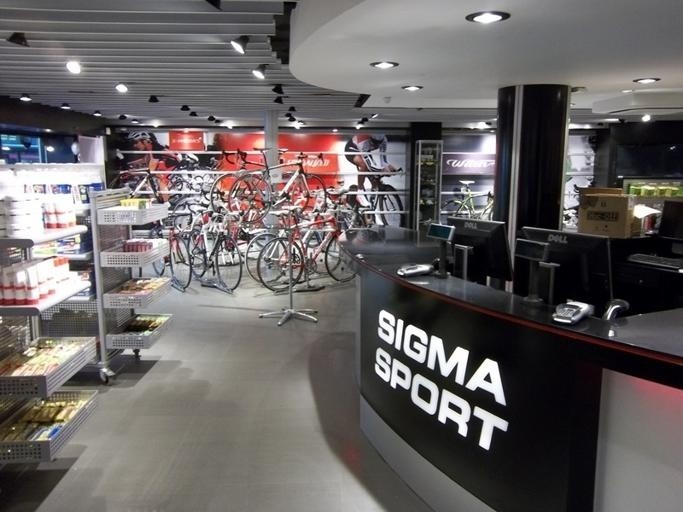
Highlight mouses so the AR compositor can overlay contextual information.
[678,268,683,273]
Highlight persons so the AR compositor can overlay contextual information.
[345,134,398,208]
[127,131,183,202]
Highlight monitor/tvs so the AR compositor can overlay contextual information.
[522,227,613,319]
[656,200,683,242]
[446,216,513,284]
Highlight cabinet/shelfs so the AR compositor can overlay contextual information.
[59,162,173,383]
[0,162,102,470]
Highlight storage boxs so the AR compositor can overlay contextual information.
[576,191,635,239]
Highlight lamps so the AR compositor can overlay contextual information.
[5,30,304,132]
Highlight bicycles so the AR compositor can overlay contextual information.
[441,181,494,219]
[347,167,404,227]
[111,147,364,292]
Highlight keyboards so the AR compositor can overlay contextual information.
[627,253,683,269]
